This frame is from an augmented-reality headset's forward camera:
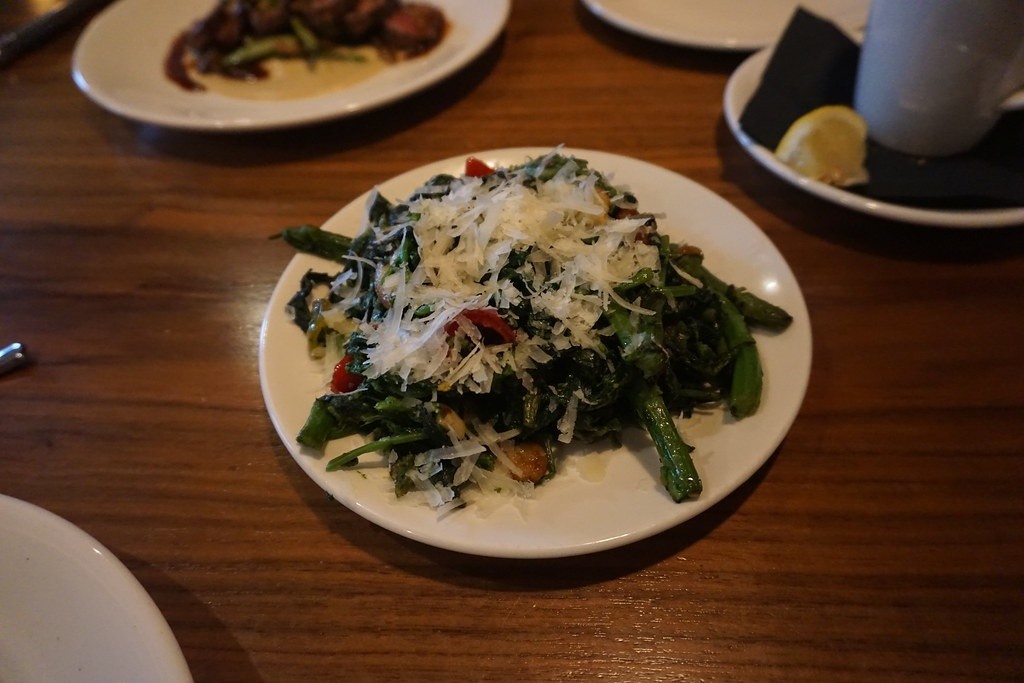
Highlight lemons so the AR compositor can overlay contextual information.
[776,106,867,187]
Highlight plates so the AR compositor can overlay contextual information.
[722,34,1024,228]
[0,493,196,683]
[257,145,815,560]
[582,0,871,52]
[70,0,512,133]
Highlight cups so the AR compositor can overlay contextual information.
[856,0,1024,169]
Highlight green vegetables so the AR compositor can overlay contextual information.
[280,160,790,502]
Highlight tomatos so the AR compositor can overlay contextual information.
[445,311,513,348]
[331,355,359,393]
